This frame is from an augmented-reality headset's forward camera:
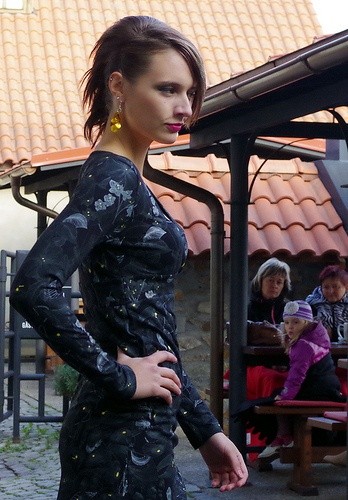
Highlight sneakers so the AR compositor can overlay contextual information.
[257,440,295,464]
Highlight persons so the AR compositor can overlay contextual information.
[223,256,297,464]
[10,14,248,500]
[255,299,340,464]
[305,266,348,398]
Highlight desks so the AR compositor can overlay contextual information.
[248,345,348,366]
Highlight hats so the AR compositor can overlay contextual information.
[283,300,313,323]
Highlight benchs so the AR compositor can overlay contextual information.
[249,401,348,497]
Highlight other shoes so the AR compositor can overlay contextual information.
[323,450,348,468]
